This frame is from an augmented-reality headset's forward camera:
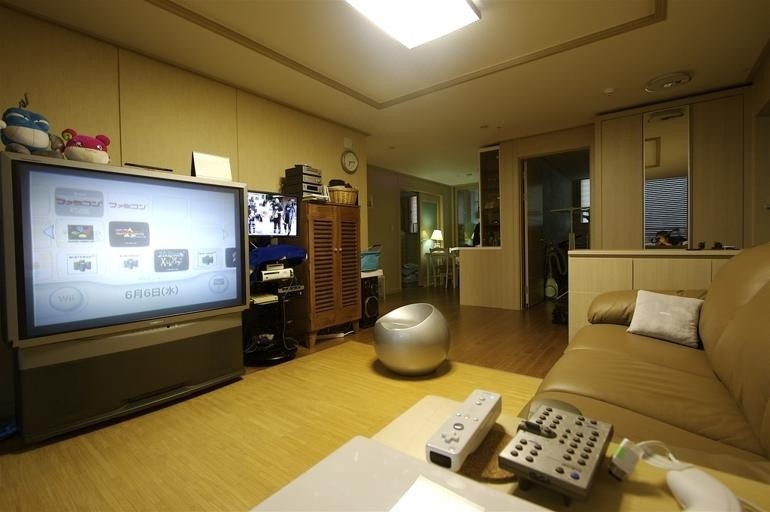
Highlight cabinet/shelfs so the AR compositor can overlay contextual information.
[247,276,308,372]
[566,93,757,344]
[279,204,362,349]
[476,145,502,248]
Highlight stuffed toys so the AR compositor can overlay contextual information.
[0,91,62,156]
[60,127,112,165]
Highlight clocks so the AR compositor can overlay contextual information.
[339,149,360,175]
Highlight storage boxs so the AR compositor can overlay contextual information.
[359,249,381,272]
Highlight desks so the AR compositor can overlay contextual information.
[249,391,770,509]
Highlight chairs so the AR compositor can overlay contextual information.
[427,247,456,289]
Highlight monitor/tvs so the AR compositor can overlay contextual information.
[248,191,299,246]
[0,151,250,349]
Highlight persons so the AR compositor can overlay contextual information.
[247,194,296,236]
[654,230,673,246]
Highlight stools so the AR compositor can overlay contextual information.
[371,300,451,378]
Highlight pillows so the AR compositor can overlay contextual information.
[626,283,705,352]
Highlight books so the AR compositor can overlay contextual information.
[251,293,278,304]
[303,185,331,203]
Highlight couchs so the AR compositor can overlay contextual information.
[516,244,770,485]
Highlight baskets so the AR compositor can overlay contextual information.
[327,187,360,206]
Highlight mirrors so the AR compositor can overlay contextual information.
[642,103,691,250]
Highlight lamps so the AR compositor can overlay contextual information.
[430,229,445,246]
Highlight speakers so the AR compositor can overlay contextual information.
[359,276,379,328]
